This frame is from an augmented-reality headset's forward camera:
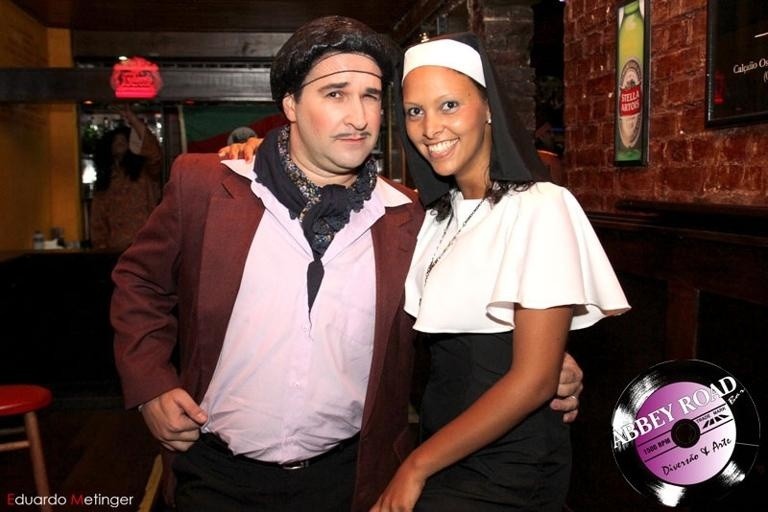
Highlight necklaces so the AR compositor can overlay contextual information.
[423,193,485,284]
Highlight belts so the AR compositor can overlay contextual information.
[251,452,334,471]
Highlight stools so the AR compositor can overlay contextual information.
[0,381,54,512]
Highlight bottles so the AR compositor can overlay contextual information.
[618,3,645,161]
[82,110,164,151]
[33,231,43,249]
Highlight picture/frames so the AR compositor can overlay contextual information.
[704,2,768,131]
[611,1,651,171]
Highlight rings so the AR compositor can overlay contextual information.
[566,395,577,401]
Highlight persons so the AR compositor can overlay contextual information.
[218,30,633,512]
[110,12,585,512]
[90,104,166,251]
